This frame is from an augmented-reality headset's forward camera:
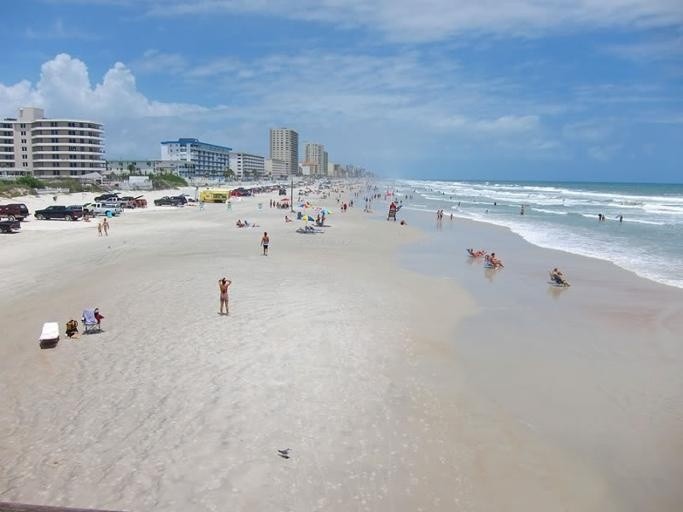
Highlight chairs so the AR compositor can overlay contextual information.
[81,307,103,334]
[39,321,58,347]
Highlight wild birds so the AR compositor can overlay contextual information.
[278,448,292,456]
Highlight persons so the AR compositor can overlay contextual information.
[102,218,110,236]
[490,253,504,268]
[436,210,440,219]
[439,210,443,219]
[557,272,570,287]
[97,223,102,237]
[486,255,500,262]
[470,248,486,255]
[217,278,231,315]
[235,194,407,235]
[552,268,565,278]
[65,319,81,339]
[260,232,269,256]
[598,214,605,222]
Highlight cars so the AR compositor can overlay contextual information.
[230,184,279,197]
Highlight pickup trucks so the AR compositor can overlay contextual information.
[0,191,231,235]
